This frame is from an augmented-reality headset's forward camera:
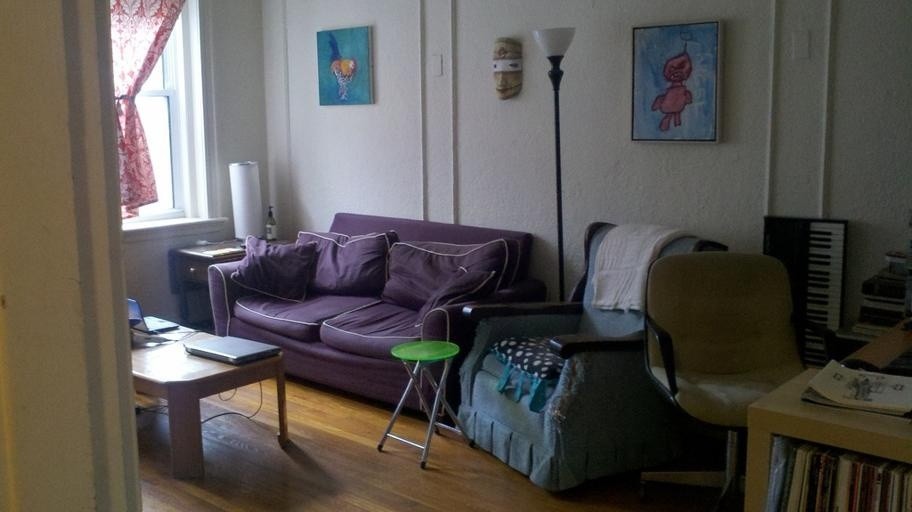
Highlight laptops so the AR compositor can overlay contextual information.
[183,335,282,366]
[127,298,179,334]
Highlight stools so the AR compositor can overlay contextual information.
[377,340,475,469]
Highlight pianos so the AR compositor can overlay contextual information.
[763,215,847,367]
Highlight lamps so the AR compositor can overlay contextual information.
[533,26,576,301]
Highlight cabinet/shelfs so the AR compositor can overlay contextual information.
[743,314,912,512]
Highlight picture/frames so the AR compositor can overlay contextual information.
[631,18,724,145]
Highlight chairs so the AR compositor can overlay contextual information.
[647,252,805,499]
[455,221,730,494]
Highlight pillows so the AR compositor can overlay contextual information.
[230,230,510,311]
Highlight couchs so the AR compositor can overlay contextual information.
[205,213,546,426]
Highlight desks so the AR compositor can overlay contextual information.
[133,315,291,481]
[173,238,296,328]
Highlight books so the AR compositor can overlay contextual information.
[765,435,912,512]
[800,359,912,416]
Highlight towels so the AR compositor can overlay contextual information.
[591,221,698,311]
